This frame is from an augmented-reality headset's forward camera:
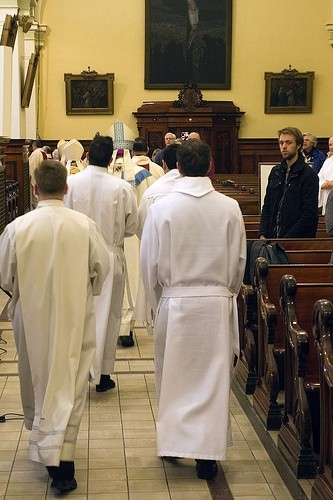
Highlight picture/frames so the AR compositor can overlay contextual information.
[64,71,114,115]
[144,0,232,91]
[265,65,315,113]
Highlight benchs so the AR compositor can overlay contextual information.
[211,173,333,500]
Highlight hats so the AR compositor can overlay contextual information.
[63,139,84,162]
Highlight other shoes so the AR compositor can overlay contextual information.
[197,464,218,479]
[51,479,77,490]
[158,456,181,460]
[122,339,134,347]
[96,379,115,392]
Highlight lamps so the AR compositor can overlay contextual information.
[12,14,32,32]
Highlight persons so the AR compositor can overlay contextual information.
[0,160,110,490]
[139,138,247,480]
[28,131,216,348]
[317,136,333,215]
[301,133,326,174]
[63,135,138,391]
[259,127,319,240]
